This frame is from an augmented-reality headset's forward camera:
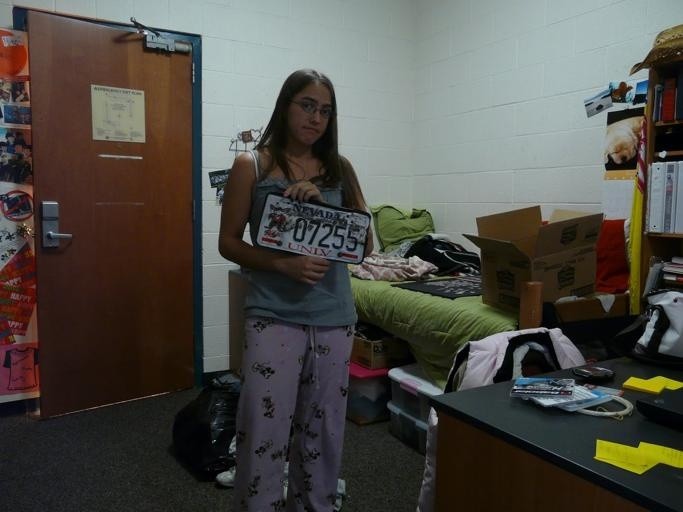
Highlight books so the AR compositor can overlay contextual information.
[510,377,614,412]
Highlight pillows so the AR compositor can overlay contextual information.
[370,202,438,252]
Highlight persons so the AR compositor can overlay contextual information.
[217,68,374,511]
[0,87,33,188]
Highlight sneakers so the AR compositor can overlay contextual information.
[214,464,237,488]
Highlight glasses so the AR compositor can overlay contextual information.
[288,97,335,120]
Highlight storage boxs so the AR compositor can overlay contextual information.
[344,326,450,459]
[456,201,610,317]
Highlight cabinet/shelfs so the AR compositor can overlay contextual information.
[227,267,249,380]
[635,59,682,316]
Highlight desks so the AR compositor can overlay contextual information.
[427,350,682,512]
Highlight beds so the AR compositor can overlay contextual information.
[348,245,631,389]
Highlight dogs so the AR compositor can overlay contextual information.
[599,115,644,164]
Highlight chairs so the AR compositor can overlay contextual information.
[456,326,562,394]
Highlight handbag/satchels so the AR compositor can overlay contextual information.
[612,285,683,366]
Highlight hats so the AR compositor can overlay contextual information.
[627,23,683,75]
